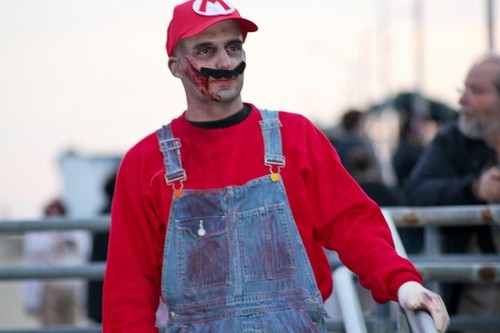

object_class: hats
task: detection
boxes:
[166,0,258,58]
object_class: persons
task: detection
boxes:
[401,57,500,316]
[389,114,427,251]
[331,110,399,206]
[85,170,118,322]
[21,200,92,329]
[100,0,451,333]
